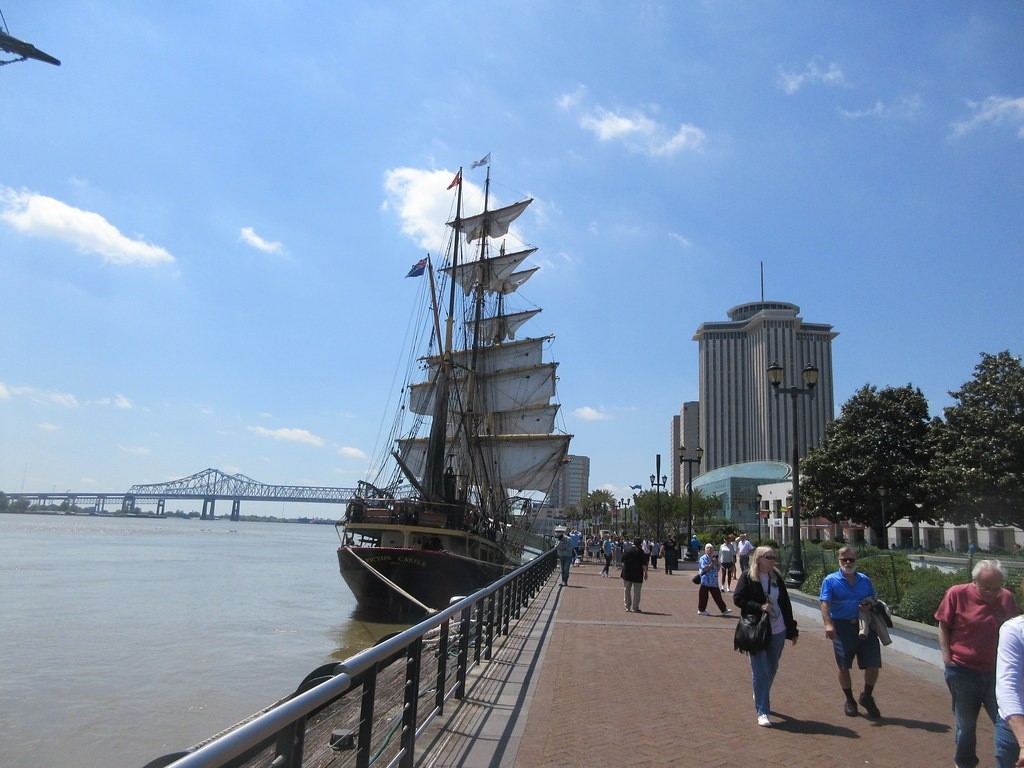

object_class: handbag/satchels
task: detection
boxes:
[734,614,772,652]
[692,574,701,584]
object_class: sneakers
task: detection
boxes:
[758,714,771,725]
[859,693,880,717]
[752,695,755,700]
[845,701,858,715]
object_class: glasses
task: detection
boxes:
[839,559,854,562]
[761,555,776,560]
[977,579,1000,593]
[712,555,719,558]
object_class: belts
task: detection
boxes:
[832,618,859,624]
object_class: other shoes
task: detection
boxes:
[605,576,610,578]
[728,588,732,592]
[697,610,709,615]
[625,609,630,612]
[559,583,568,586]
[721,609,732,615]
[633,610,641,612]
[599,572,604,577]
[721,588,724,592]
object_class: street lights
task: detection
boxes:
[766,359,819,590]
[756,491,762,541]
[612,501,621,535]
[621,497,630,538]
[633,493,643,537]
[677,444,704,562]
[650,474,668,546]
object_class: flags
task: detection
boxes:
[447,173,459,190]
[471,154,488,170]
[405,258,427,278]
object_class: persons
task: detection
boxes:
[598,534,612,578]
[969,539,976,553]
[818,548,882,719]
[718,534,754,593]
[691,535,700,560]
[554,534,573,586]
[569,530,660,569]
[733,546,799,727]
[934,559,1021,768]
[663,533,678,575]
[698,544,732,615]
[620,537,648,613]
[995,613,1024,768]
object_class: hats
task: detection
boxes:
[693,535,696,538]
[558,534,563,541]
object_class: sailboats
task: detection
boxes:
[333,152,574,622]
